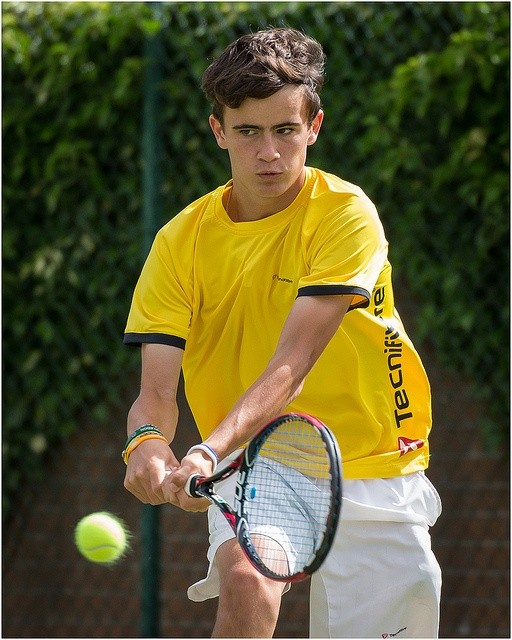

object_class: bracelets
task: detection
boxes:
[185,444,220,474]
[119,424,169,465]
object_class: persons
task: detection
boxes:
[121,28,443,637]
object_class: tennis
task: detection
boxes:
[73,512,128,563]
